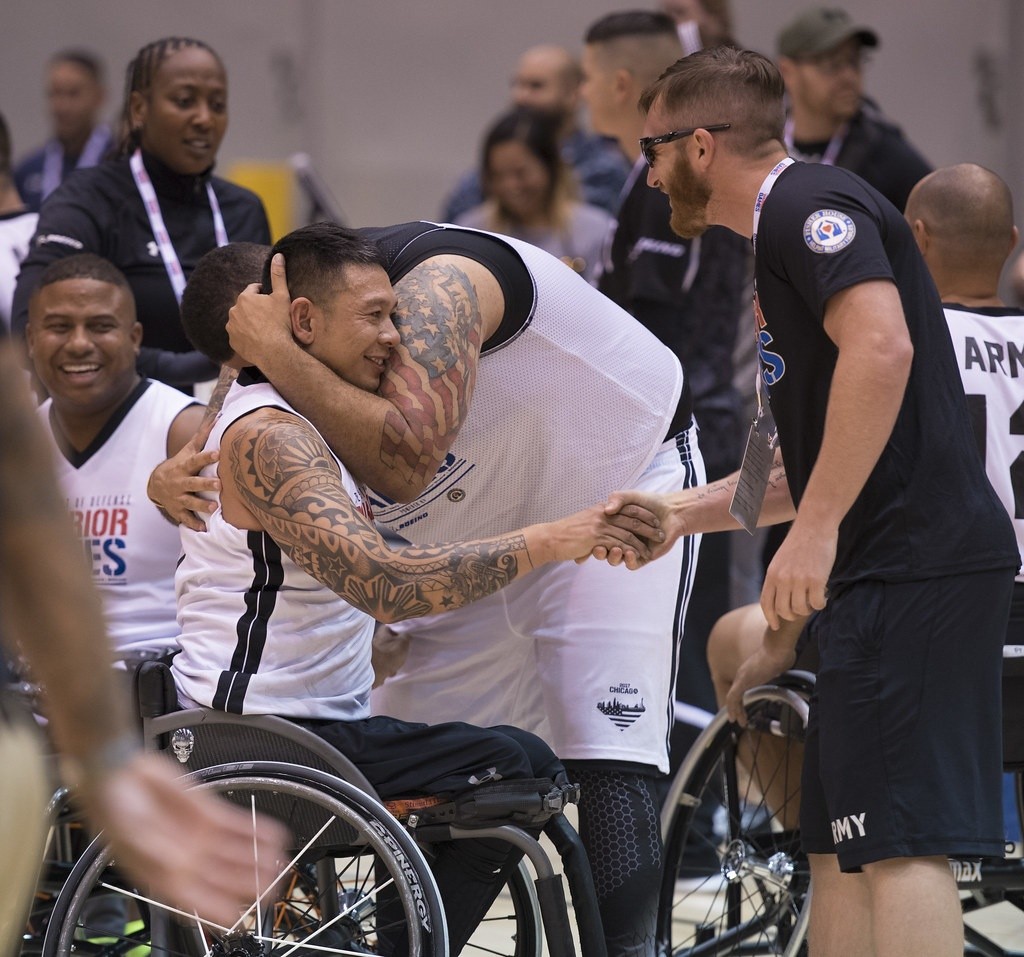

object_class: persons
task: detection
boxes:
[440,0,1024,957]
[0,37,709,957]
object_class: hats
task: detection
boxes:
[781,6,878,58]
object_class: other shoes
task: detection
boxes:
[745,815,808,866]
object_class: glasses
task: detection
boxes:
[640,120,732,167]
[815,52,871,72]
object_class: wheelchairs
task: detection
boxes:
[46,650,606,956]
[653,664,1023,957]
